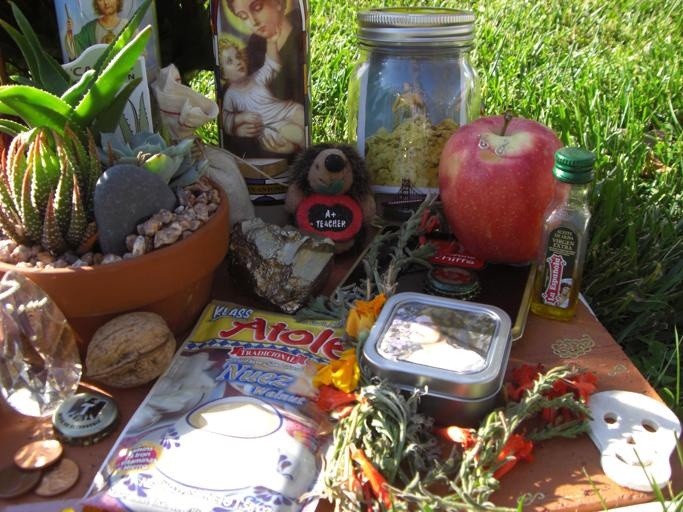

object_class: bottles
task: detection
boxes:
[528,146,597,321]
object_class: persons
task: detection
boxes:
[217,22,306,146]
[63,1,149,63]
[217,0,305,158]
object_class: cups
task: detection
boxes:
[151,396,315,498]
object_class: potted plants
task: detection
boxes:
[0,1,230,344]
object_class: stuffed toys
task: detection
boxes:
[280,140,377,264]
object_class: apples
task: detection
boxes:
[439,115,569,269]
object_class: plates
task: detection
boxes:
[107,423,320,512]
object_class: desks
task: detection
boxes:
[0,155,681,512]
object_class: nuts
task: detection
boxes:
[83,311,177,388]
